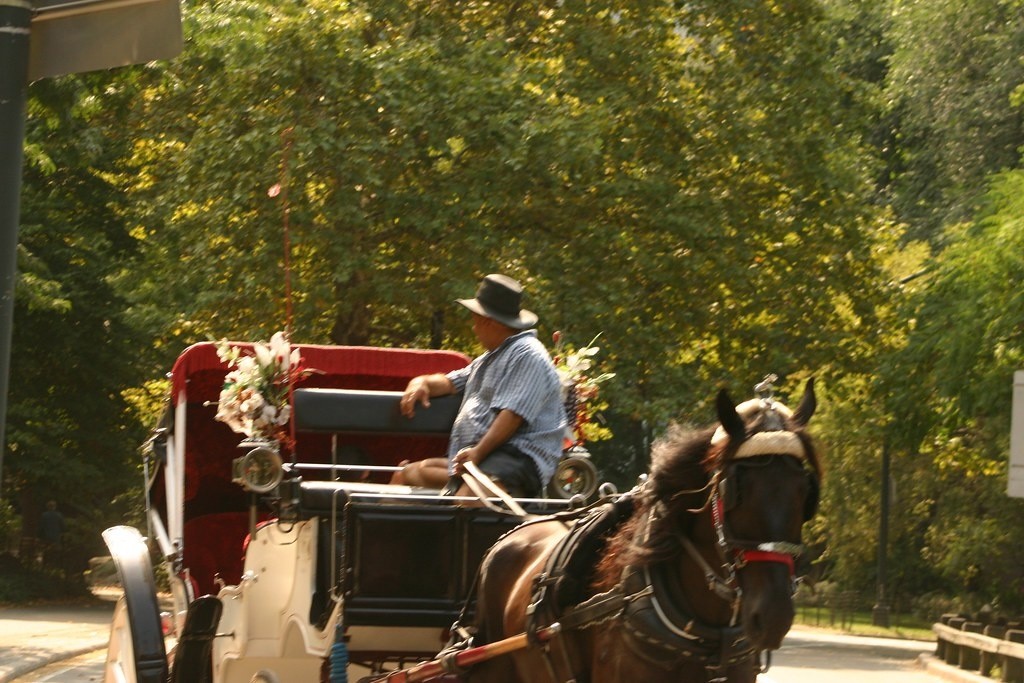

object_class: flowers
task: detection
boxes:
[550,330,620,448]
[206,328,301,454]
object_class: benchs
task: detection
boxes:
[292,387,557,611]
[176,510,276,604]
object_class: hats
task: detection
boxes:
[456,273,539,330]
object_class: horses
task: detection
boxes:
[447,374,828,683]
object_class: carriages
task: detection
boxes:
[101,340,821,683]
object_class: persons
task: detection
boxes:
[333,440,410,503]
[403,275,569,509]
